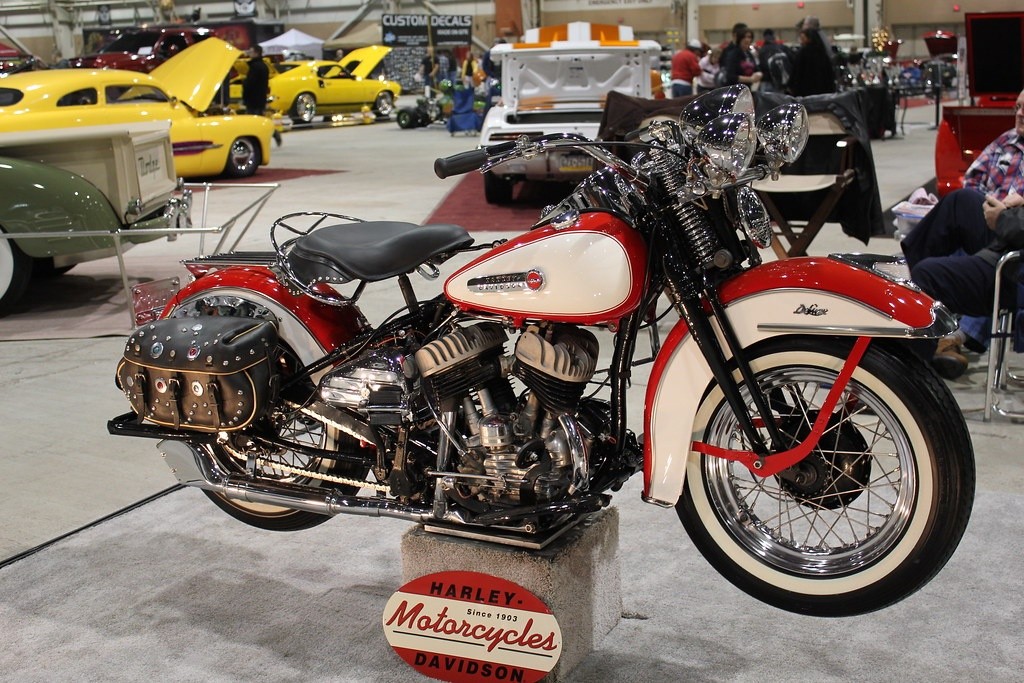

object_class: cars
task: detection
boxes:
[479,20,667,206]
[0,36,282,180]
[0,118,194,317]
[227,45,402,124]
[71,27,216,75]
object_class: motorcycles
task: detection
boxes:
[106,84,978,618]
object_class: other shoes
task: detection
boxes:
[273,130,281,146]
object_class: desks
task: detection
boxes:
[891,83,941,135]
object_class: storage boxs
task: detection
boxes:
[115,317,280,433]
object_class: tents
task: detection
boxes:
[258,23,382,62]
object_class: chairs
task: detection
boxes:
[982,249,1024,422]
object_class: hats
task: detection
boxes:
[688,38,702,48]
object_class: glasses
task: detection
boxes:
[1015,105,1024,112]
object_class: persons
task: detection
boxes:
[336,49,344,62]
[205,44,269,116]
[413,38,508,106]
[670,15,847,98]
[900,89,1024,380]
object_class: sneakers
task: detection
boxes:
[934,335,968,381]
